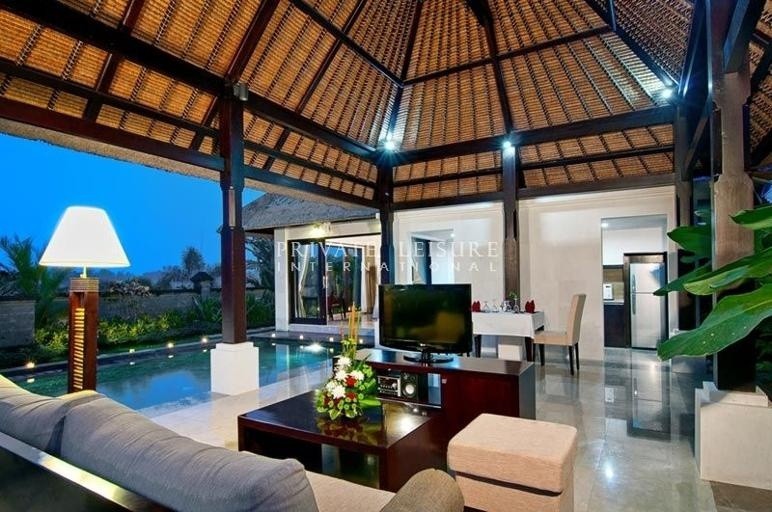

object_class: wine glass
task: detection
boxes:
[482,298,520,313]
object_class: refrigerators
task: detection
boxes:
[629,262,667,350]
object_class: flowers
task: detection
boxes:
[311,301,380,420]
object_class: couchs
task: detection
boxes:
[1,375,465,512]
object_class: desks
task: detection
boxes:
[332,347,536,447]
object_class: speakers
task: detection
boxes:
[238,83,248,101]
[400,371,429,401]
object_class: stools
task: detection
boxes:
[447,414,578,511]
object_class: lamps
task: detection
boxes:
[39,207,131,292]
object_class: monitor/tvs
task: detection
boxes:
[378,283,473,364]
